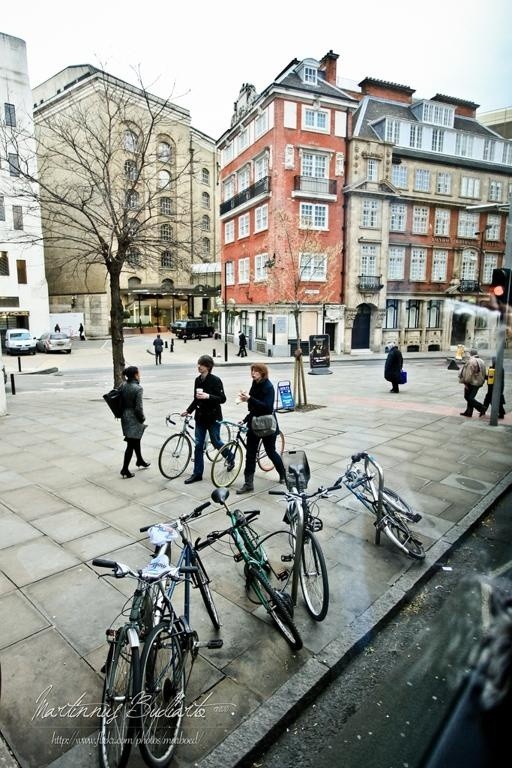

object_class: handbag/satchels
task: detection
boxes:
[103,389,126,418]
[401,371,406,384]
[251,415,277,438]
[156,345,163,352]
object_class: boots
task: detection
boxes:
[236,469,255,494]
[275,462,286,484]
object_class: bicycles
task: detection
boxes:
[158,411,231,478]
[212,417,285,486]
[89,450,426,768]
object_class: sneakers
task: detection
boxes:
[460,405,504,418]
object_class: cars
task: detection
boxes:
[36,331,71,353]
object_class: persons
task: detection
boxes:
[118,366,151,479]
[181,355,235,483]
[238,331,247,356]
[480,357,505,419]
[79,323,85,340]
[384,343,403,393]
[235,363,286,494]
[153,335,163,364]
[55,324,60,332]
[459,348,487,417]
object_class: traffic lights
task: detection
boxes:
[491,268,512,302]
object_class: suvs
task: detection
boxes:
[173,318,214,339]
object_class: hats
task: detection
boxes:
[469,349,477,355]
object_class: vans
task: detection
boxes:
[4,327,36,355]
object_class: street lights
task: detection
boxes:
[474,224,492,304]
[223,259,232,362]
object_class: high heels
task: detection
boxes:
[120,470,135,478]
[136,462,150,467]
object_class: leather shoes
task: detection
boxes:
[185,475,202,484]
[227,455,234,472]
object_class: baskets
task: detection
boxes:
[281,450,311,489]
